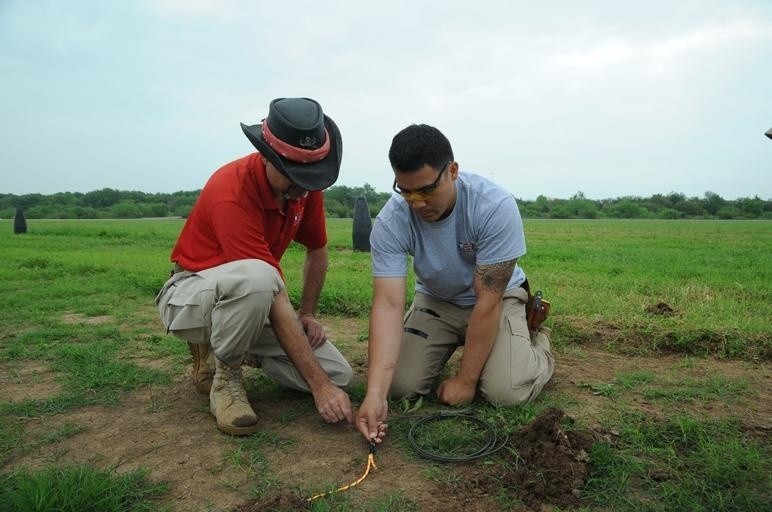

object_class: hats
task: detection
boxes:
[240,96,343,192]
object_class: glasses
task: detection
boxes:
[393,162,450,201]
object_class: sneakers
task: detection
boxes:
[536,326,554,344]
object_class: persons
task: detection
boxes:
[157,96,354,437]
[354,122,558,445]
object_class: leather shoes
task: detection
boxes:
[209,361,259,435]
[189,335,215,394]
